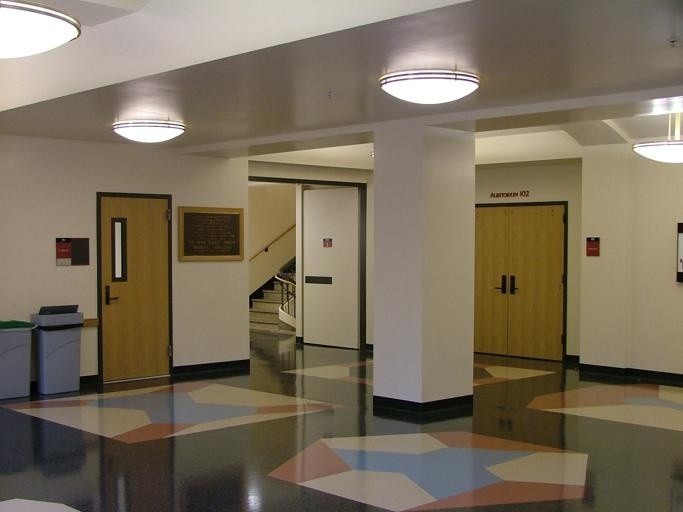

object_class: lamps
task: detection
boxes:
[0,0,81,60]
[112,119,186,144]
[377,68,480,105]
[631,111,683,164]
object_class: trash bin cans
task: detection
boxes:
[30,304,84,394]
[0,320,38,399]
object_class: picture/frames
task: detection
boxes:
[178,206,244,263]
